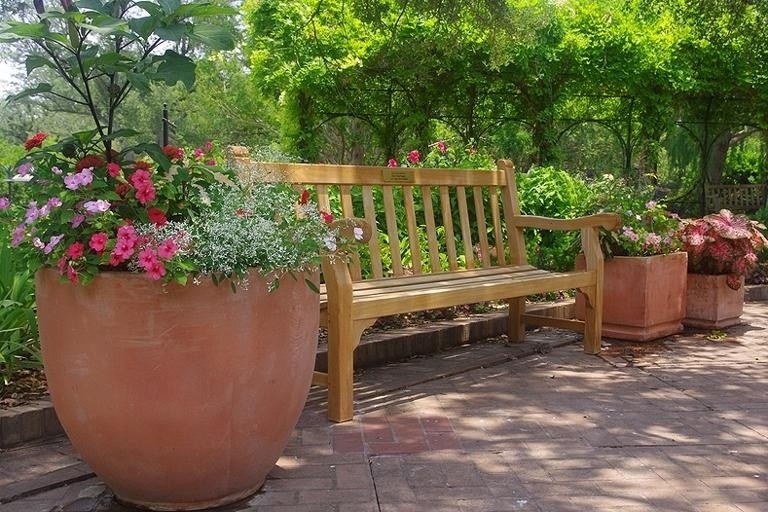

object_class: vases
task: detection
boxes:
[32,260,319,511]
[575,251,746,342]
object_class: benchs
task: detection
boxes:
[223,143,622,424]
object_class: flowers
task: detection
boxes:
[0,128,364,297]
[581,173,768,291]
